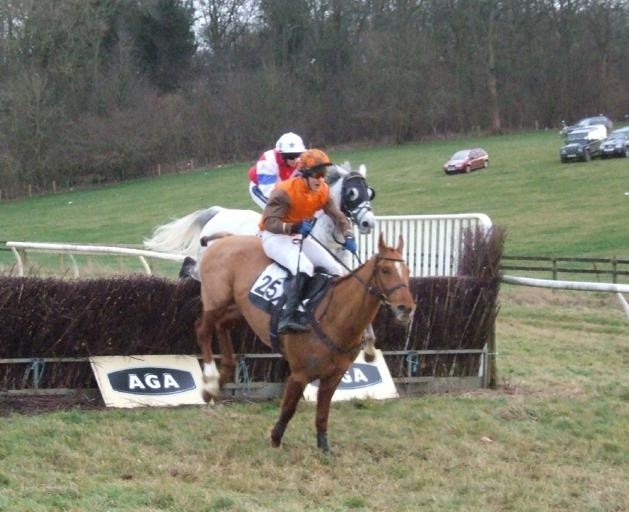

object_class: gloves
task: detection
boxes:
[291,219,311,236]
[343,236,356,253]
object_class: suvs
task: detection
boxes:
[559,116,629,163]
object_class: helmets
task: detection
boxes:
[297,149,332,172]
[276,132,305,153]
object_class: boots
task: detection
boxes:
[278,273,310,333]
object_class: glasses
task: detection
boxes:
[310,168,327,178]
[283,153,299,160]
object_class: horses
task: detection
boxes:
[142,160,377,364]
[160,232,417,455]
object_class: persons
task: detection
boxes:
[247,131,307,215]
[258,146,359,337]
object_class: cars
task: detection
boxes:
[442,148,489,175]
